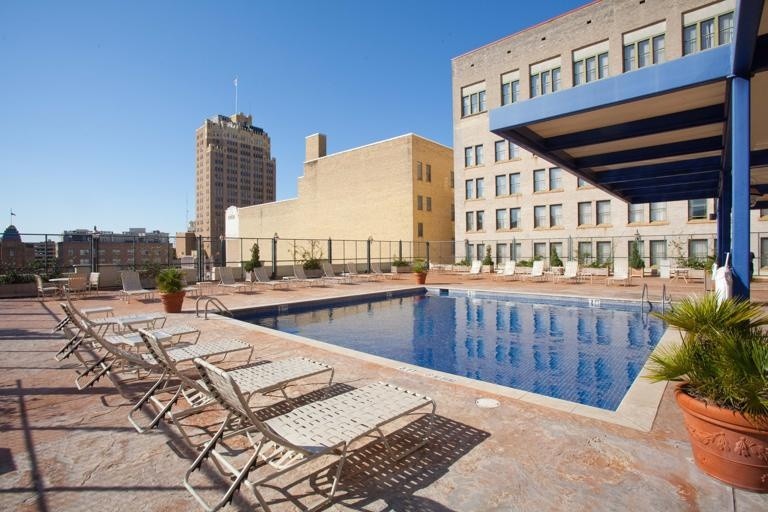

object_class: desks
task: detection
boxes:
[669,268,690,284]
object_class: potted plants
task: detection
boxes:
[703,261,715,291]
[244,243,260,283]
[639,289,768,494]
[481,255,494,273]
[551,248,564,275]
[630,238,645,278]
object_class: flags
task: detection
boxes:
[11,213,16,216]
[232,79,238,86]
